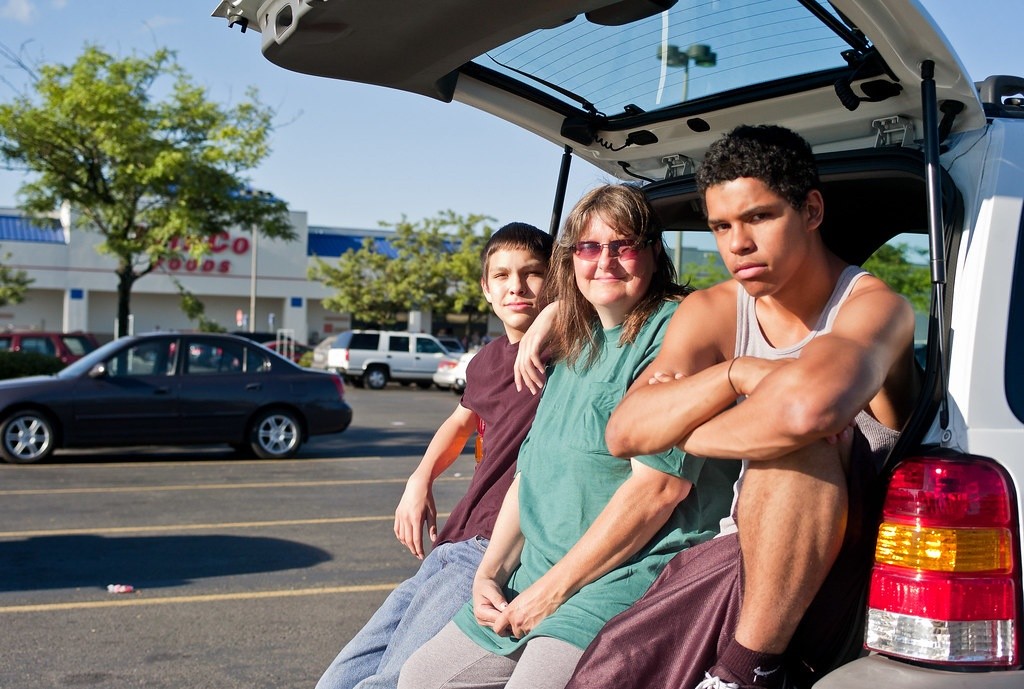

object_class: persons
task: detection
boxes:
[564,124,931,689]
[397,185,741,689]
[313,224,567,689]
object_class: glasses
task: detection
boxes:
[569,239,656,261]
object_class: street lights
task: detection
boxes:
[655,41,718,291]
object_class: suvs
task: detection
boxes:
[202,0,1021,689]
[327,329,458,391]
[1,331,105,376]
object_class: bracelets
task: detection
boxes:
[727,356,743,397]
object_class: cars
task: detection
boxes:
[432,351,482,395]
[230,339,316,370]
[1,329,354,463]
